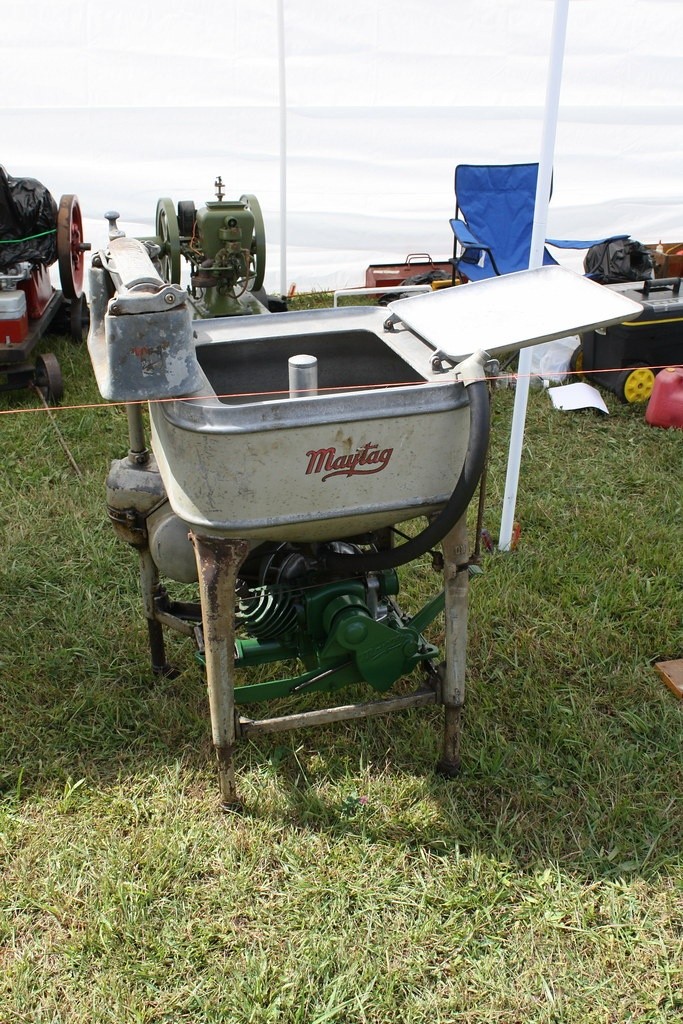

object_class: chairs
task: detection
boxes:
[448,163,631,287]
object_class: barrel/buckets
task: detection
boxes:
[645,367,683,428]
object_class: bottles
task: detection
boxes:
[656,240,665,254]
